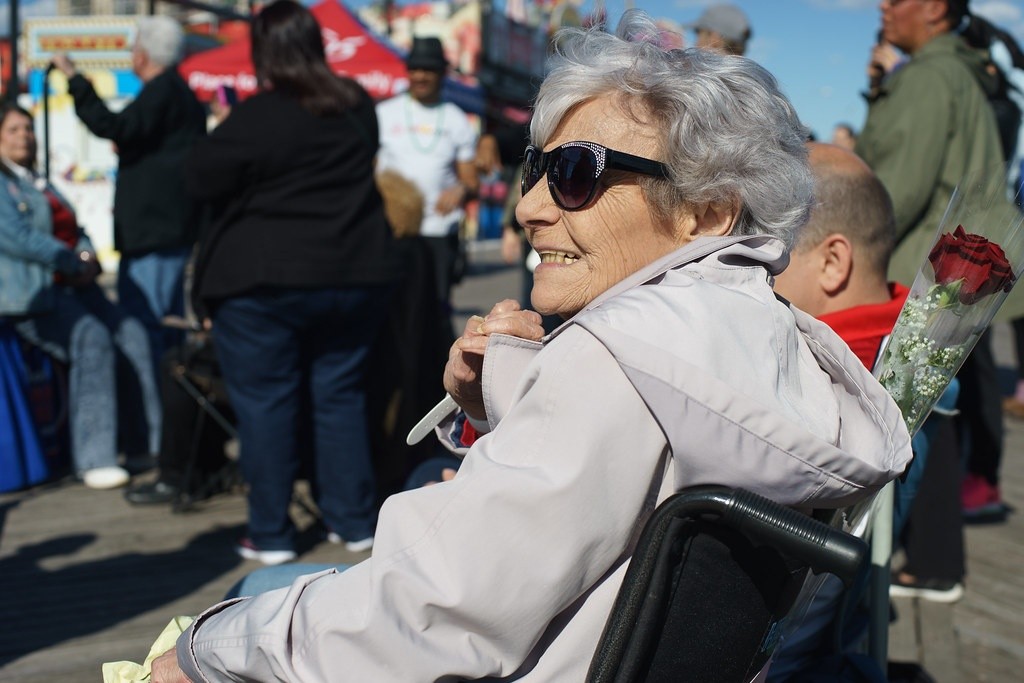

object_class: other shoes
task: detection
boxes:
[963,474,1001,514]
[327,532,374,552]
[83,465,130,490]
[888,567,962,603]
[123,458,236,511]
[233,528,296,564]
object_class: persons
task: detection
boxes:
[158,12,907,683]
[2,1,1024,601]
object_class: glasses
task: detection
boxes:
[520,140,669,211]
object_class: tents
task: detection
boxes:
[170,0,487,114]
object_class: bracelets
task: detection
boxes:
[459,182,473,201]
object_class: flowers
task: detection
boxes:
[880,228,1018,434]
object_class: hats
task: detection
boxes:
[684,3,751,44]
[403,37,448,72]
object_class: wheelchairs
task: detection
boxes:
[580,481,872,683]
